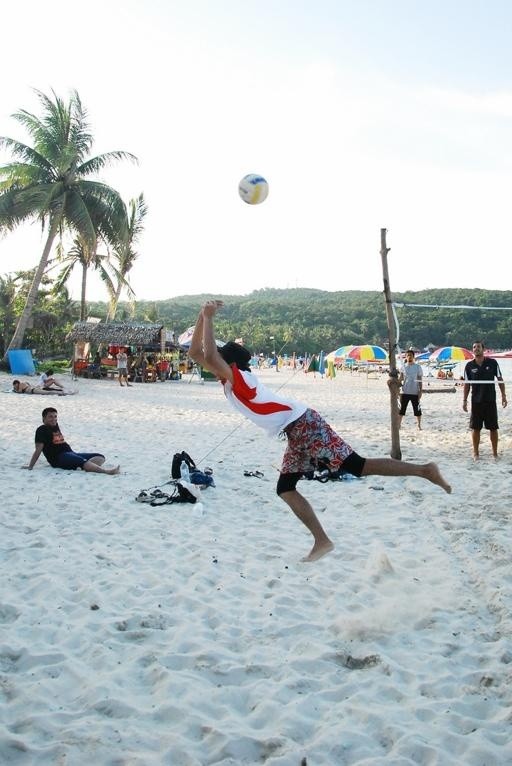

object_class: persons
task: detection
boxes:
[36,370,66,389]
[19,406,121,475]
[396,350,423,430]
[187,299,454,564]
[93,352,104,379]
[116,346,133,387]
[461,341,508,463]
[11,380,64,396]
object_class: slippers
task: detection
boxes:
[136,489,170,506]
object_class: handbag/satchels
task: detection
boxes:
[171,451,196,479]
[190,472,215,489]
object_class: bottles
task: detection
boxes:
[180,460,190,482]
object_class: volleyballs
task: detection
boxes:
[238,173,268,203]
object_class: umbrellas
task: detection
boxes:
[269,343,474,379]
